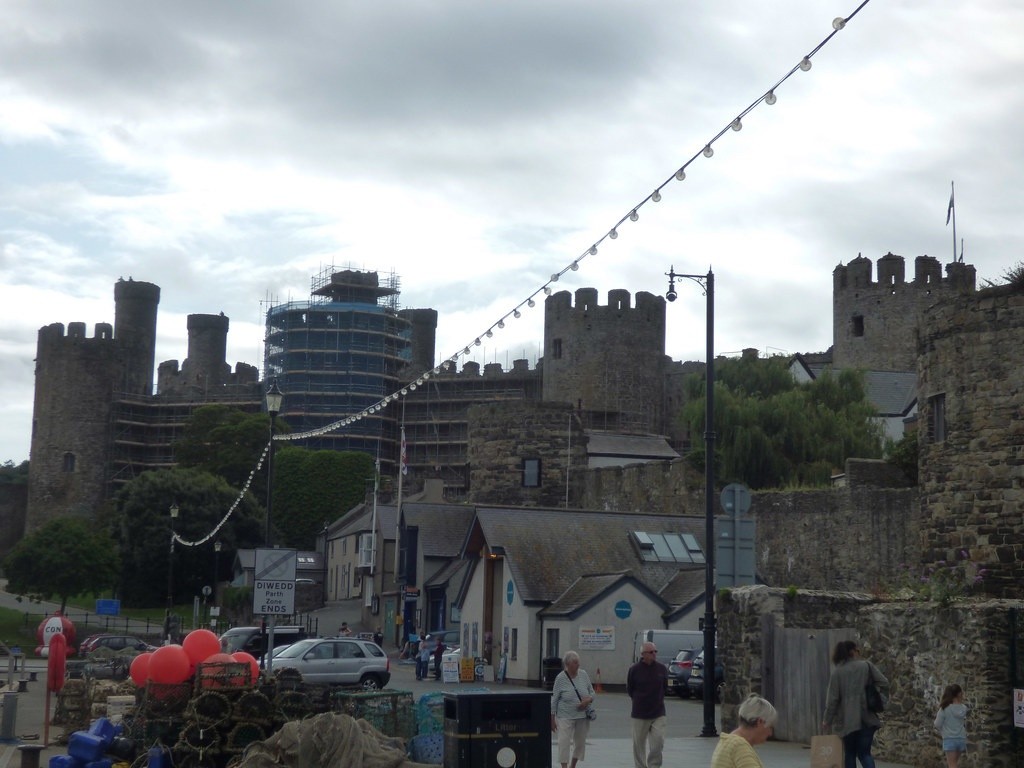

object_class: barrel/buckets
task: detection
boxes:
[50,718,171,768]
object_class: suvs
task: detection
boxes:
[218,625,311,662]
[686,644,723,703]
[667,648,702,699]
[79,633,160,657]
[256,638,391,691]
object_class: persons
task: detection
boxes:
[431,637,444,680]
[415,632,431,680]
[934,684,968,768]
[822,641,890,768]
[710,697,778,768]
[627,642,668,768]
[374,625,383,648]
[551,652,595,768]
[339,622,352,637]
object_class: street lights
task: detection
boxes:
[261,377,285,636]
[164,499,181,640]
[662,262,723,739]
[212,539,225,634]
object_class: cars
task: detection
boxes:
[414,630,461,677]
[254,643,291,667]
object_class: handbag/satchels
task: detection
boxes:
[423,641,429,648]
[586,708,596,720]
[811,733,845,768]
[866,662,884,713]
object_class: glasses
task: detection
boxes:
[645,650,658,654]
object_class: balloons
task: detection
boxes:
[129,630,259,697]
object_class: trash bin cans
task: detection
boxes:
[0,691,16,741]
[542,657,564,691]
[443,692,554,768]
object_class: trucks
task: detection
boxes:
[632,629,705,669]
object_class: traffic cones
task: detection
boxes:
[594,668,603,694]
